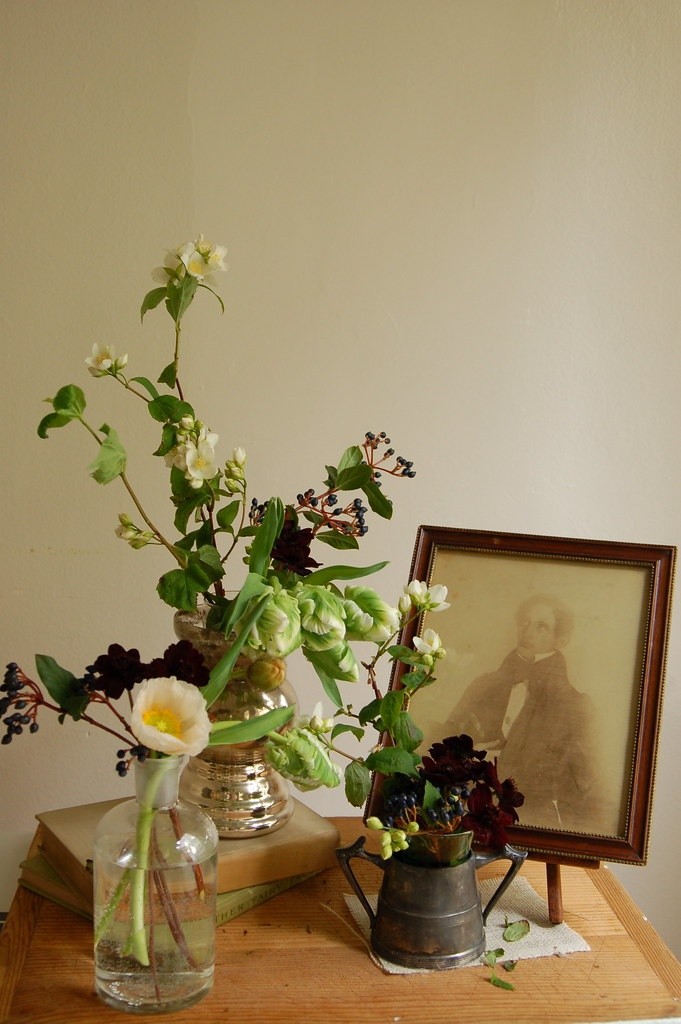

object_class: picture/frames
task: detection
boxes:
[362,523,676,865]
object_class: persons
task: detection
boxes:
[441,591,603,833]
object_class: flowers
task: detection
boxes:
[0,637,209,970]
[367,731,524,844]
[4,212,449,804]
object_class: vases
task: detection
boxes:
[173,589,293,838]
[334,828,529,969]
[89,756,218,1014]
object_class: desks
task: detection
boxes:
[4,812,679,1019]
